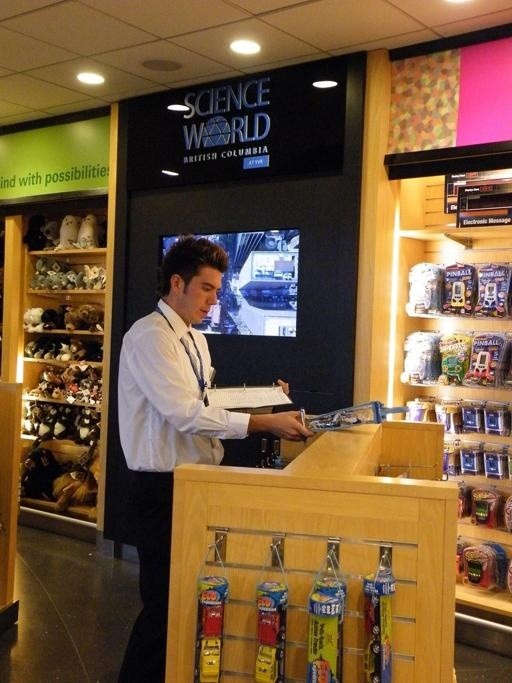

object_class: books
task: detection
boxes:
[202,381,296,415]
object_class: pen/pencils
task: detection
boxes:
[300,408,306,445]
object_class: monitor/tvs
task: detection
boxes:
[157,226,306,345]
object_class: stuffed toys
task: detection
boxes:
[19,211,105,515]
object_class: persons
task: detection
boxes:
[115,238,316,683]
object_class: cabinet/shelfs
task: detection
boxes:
[0,196,127,545]
